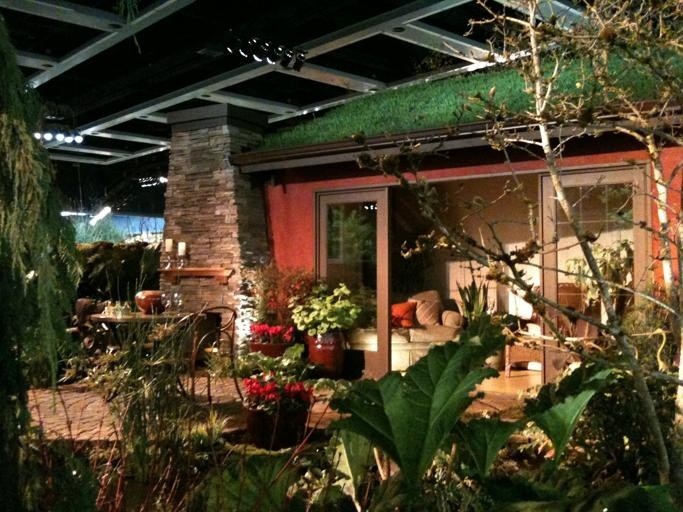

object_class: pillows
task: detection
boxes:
[387,291,445,328]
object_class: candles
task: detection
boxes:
[163,238,186,268]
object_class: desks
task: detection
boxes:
[90,310,194,407]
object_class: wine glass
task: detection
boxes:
[160,291,186,316]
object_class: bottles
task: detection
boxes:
[105,299,131,318]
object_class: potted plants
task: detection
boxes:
[287,282,360,376]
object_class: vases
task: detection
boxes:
[134,289,169,315]
[247,407,307,450]
[248,341,289,358]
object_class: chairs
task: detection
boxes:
[169,303,243,403]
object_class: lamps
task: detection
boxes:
[41,127,83,145]
[225,36,305,74]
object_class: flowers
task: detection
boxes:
[248,322,293,342]
[243,371,313,408]
